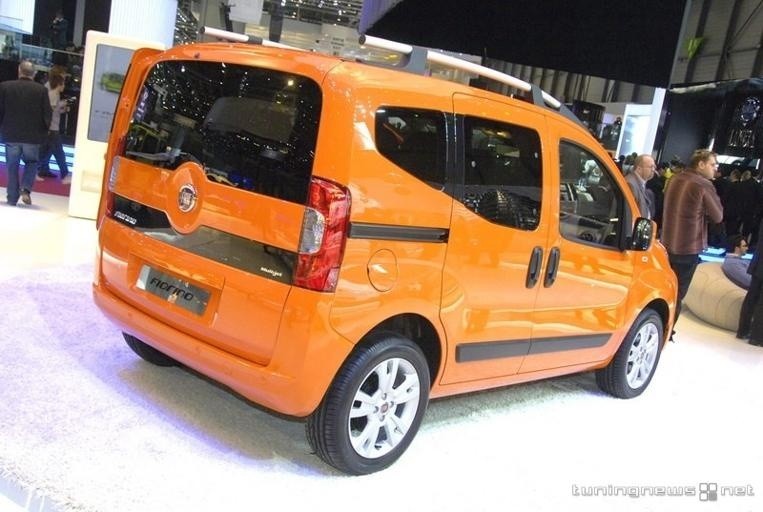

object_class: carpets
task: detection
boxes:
[0,160,72,199]
[1,278,611,512]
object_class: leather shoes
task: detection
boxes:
[735,331,763,347]
[19,188,32,205]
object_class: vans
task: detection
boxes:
[91,25,680,477]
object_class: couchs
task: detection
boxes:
[680,259,757,339]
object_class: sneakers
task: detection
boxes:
[29,170,71,185]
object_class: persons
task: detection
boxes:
[723,233,753,288]
[733,208,762,348]
[659,146,724,345]
[613,149,761,252]
[0,10,93,205]
[581,159,603,185]
